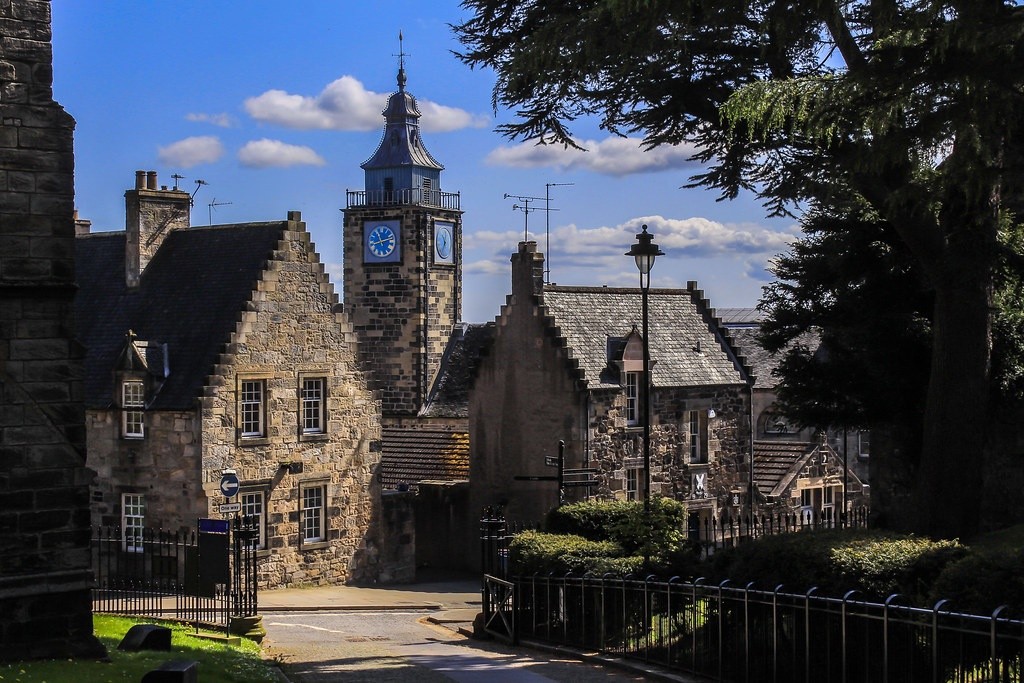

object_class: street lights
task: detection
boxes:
[624,223,666,631]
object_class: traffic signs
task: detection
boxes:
[563,480,601,487]
[563,468,598,475]
[545,456,559,467]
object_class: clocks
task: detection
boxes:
[436,227,453,259]
[367,226,397,259]
